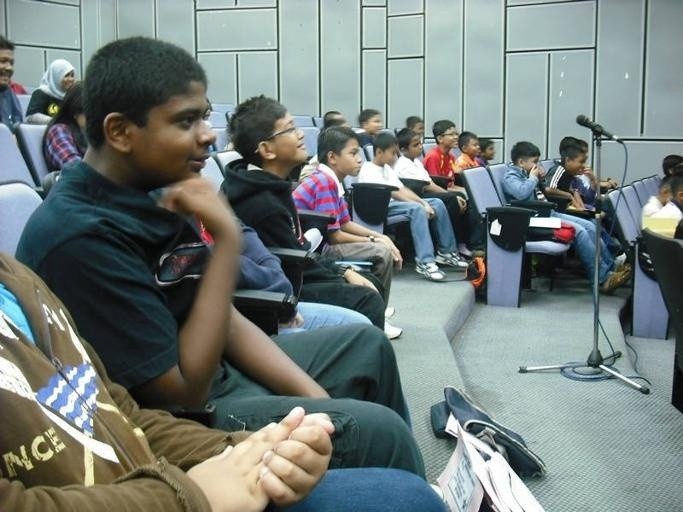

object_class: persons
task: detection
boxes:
[0,248,450,511]
[202,95,682,339]
[1,36,91,173]
[14,38,427,479]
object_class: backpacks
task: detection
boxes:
[528,218,576,243]
[427,384,552,476]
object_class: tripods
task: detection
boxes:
[516,144,651,396]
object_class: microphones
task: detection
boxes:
[576,113,624,145]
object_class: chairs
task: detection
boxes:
[619,184,643,234]
[216,149,335,254]
[209,112,228,127]
[540,159,555,173]
[210,104,234,113]
[642,226,682,415]
[460,165,571,307]
[46,172,317,335]
[351,127,395,136]
[314,116,324,128]
[363,143,454,198]
[300,126,321,155]
[17,95,31,122]
[0,123,37,189]
[199,154,373,267]
[293,115,313,129]
[226,111,235,124]
[13,124,51,186]
[342,146,430,235]
[632,180,648,207]
[487,163,574,244]
[210,127,228,152]
[423,143,436,157]
[452,149,461,157]
[605,188,669,340]
[0,181,298,428]
[642,174,662,196]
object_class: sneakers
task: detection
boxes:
[384,321,403,338]
[457,241,474,255]
[434,253,466,268]
[614,250,627,266]
[599,266,635,296]
[384,305,397,319]
[413,261,444,279]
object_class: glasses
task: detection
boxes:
[250,121,301,154]
[438,130,458,137]
